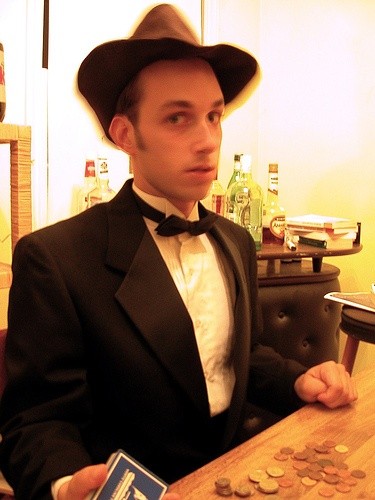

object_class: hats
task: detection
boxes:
[77,4,261,145]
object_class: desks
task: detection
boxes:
[159,371,375,500]
[241,244,363,444]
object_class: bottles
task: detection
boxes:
[205,169,225,218]
[226,154,241,221]
[87,158,116,209]
[264,163,285,246]
[77,160,98,214]
[231,156,262,251]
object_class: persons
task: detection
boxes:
[1,4,359,500]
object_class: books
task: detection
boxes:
[286,214,358,249]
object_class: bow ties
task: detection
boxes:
[153,211,219,237]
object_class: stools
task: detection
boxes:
[340,305,375,378]
